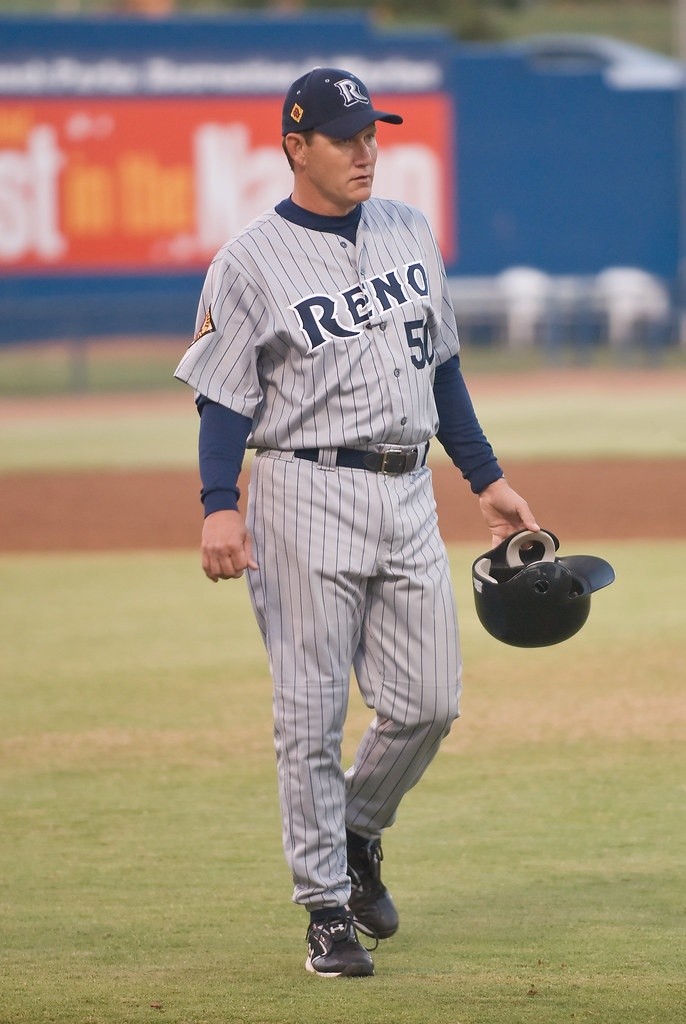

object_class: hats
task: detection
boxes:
[277,68,405,140]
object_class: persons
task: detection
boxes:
[173,68,541,978]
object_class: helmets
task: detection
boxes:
[468,525,620,649]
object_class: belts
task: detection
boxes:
[292,436,431,476]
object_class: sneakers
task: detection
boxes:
[302,903,381,979]
[344,830,402,939]
